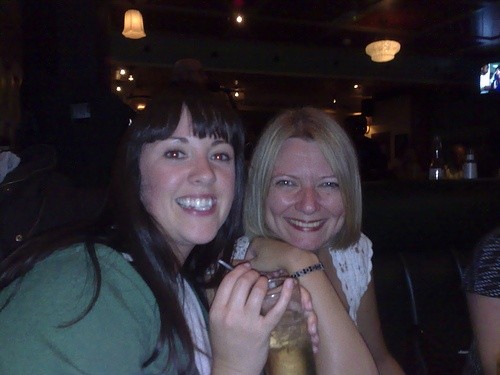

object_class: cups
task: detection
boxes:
[260,277,317,375]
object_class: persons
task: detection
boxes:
[231,107,403,375]
[0,90,319,375]
[462,226,500,375]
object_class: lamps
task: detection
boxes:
[365,17,401,62]
[122,9,146,40]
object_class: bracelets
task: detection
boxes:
[291,263,323,278]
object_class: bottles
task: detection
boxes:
[427,136,445,180]
[464,148,478,180]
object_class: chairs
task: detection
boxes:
[398,248,472,374]
